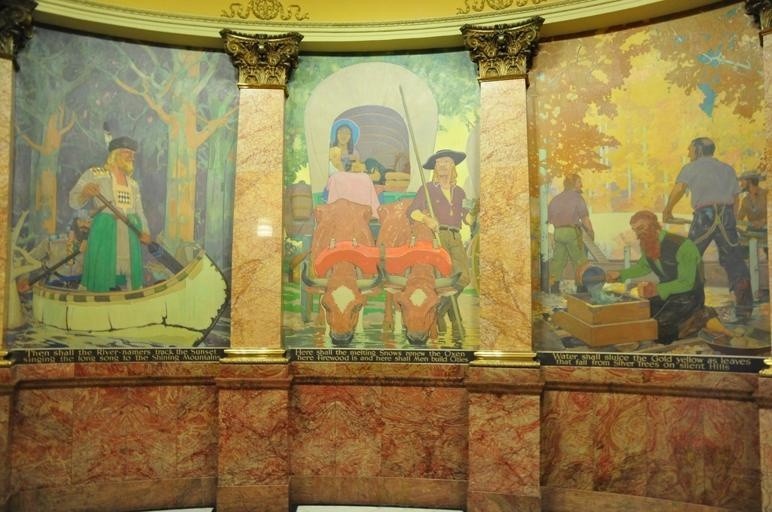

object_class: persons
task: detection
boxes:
[327,119,381,218]
[606,211,719,345]
[547,173,594,289]
[68,136,152,292]
[407,149,481,333]
[663,137,753,321]
[736,171,767,230]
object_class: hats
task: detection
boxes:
[421,147,468,170]
[330,118,361,146]
[735,169,767,181]
[109,135,138,153]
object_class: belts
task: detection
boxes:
[439,224,460,233]
[553,223,576,229]
